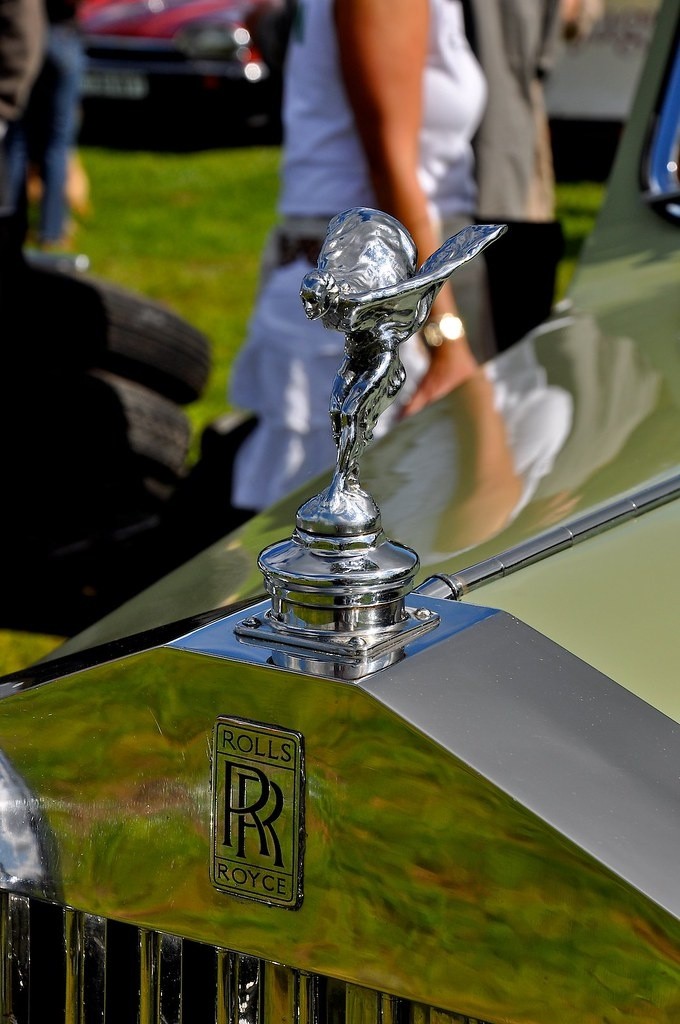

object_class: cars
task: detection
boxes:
[49,1,299,154]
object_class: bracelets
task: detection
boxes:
[420,312,467,351]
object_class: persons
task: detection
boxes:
[0,0,88,258]
[233,0,490,513]
